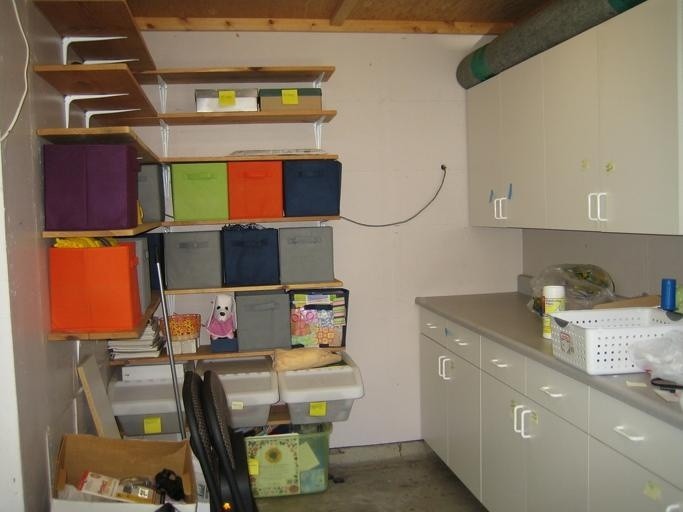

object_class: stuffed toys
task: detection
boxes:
[199,295,237,341]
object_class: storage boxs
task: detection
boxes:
[275,348,363,423]
[236,422,334,498]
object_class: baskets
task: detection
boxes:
[159,314,201,341]
[549,307,683,376]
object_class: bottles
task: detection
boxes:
[539,284,565,342]
[660,278,676,312]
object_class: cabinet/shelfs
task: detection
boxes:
[586,388,681,512]
[541,0,681,236]
[478,334,585,512]
[417,304,480,503]
[465,49,541,228]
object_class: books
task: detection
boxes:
[105,315,165,362]
